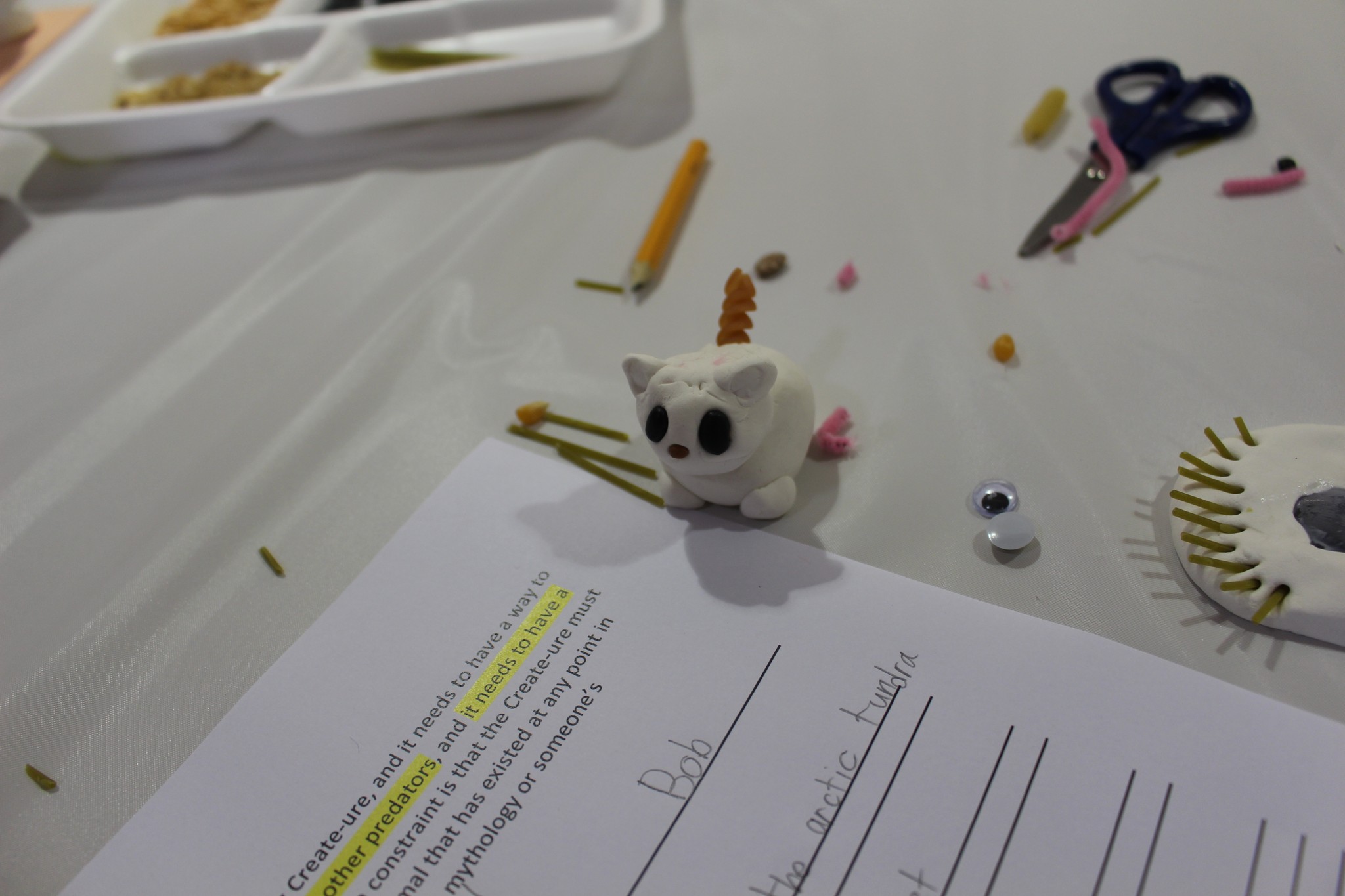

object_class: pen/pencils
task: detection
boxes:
[627,139,709,290]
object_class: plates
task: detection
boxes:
[2,0,673,157]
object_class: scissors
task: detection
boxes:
[1018,56,1255,257]
